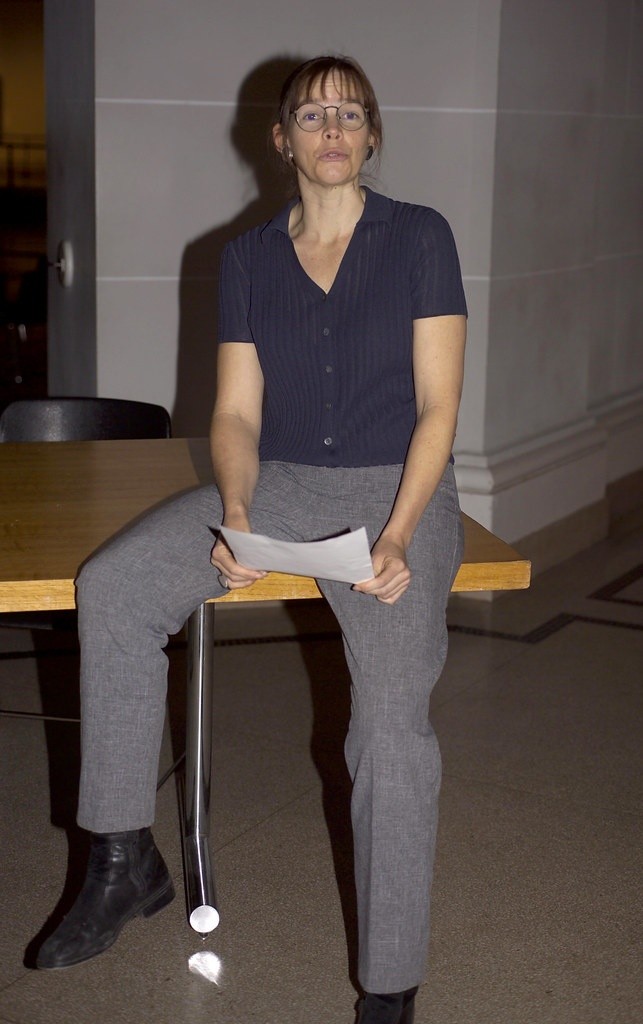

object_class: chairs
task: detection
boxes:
[2,399,186,796]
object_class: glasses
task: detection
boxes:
[286,101,370,132]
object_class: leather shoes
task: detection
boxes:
[35,830,177,971]
[354,985,418,1023]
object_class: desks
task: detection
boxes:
[0,437,533,936]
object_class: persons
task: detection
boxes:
[32,54,473,1024]
